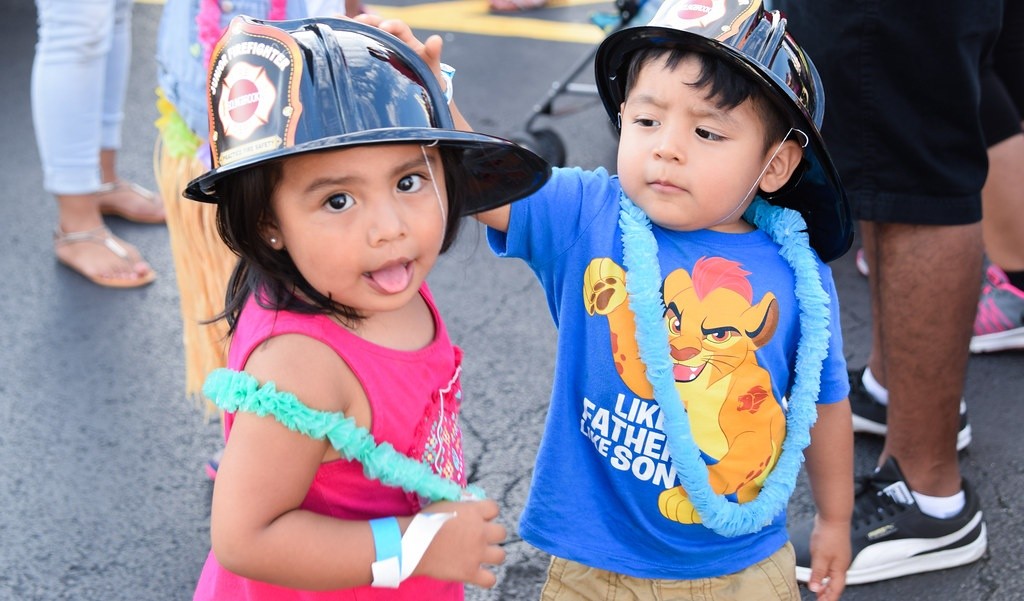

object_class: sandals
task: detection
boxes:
[98,179,166,223]
[52,222,155,286]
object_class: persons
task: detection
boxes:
[350,1,856,600]
[153,0,366,480]
[759,0,1024,586]
[28,0,173,290]
[189,12,554,601]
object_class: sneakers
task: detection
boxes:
[790,454,988,584]
[969,263,1024,353]
[840,367,972,451]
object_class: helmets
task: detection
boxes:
[595,0,825,198]
[183,13,553,217]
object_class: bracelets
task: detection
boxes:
[440,60,456,103]
[367,505,457,589]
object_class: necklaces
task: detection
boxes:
[600,171,831,539]
